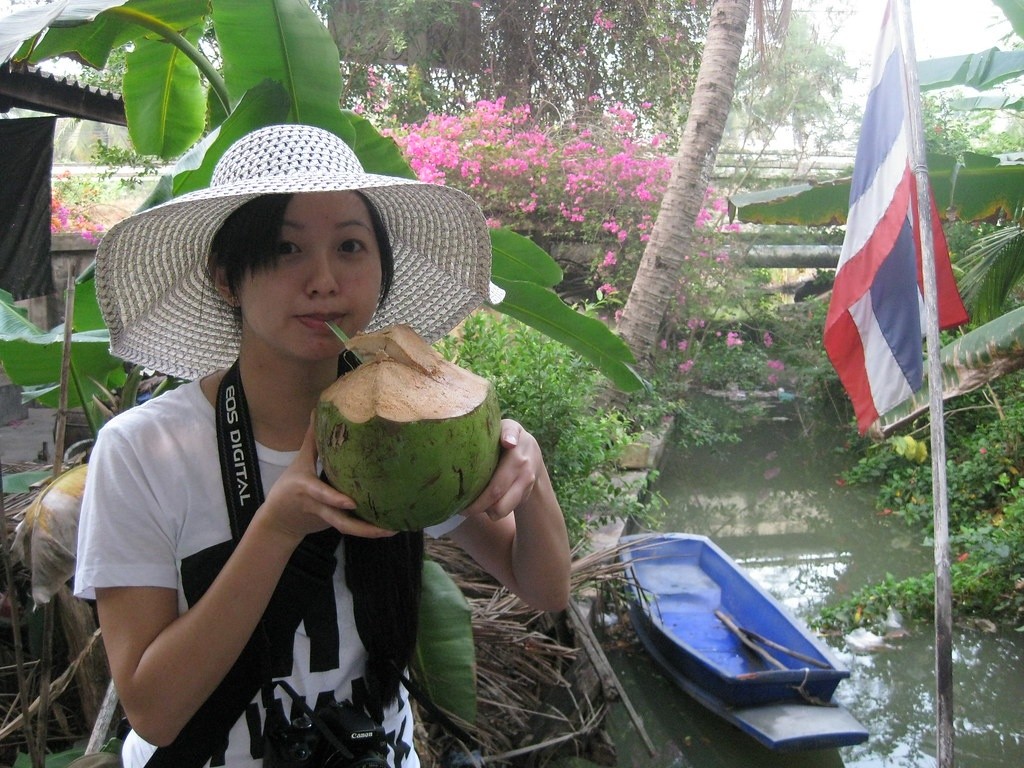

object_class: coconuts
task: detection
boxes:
[314,322,502,528]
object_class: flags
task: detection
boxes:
[823,1,970,436]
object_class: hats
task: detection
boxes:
[95,124,489,381]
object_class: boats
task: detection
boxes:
[618,532,870,753]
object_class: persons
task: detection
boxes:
[71,124,572,768]
